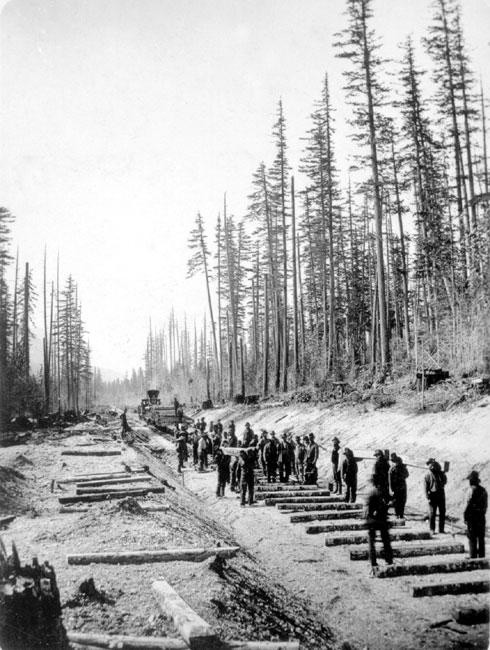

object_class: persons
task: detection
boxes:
[390,456,410,519]
[424,458,448,535]
[329,437,343,495]
[119,408,129,440]
[176,404,184,423]
[340,446,363,502]
[462,471,489,560]
[362,473,396,568]
[174,398,180,414]
[369,449,394,519]
[174,415,319,506]
